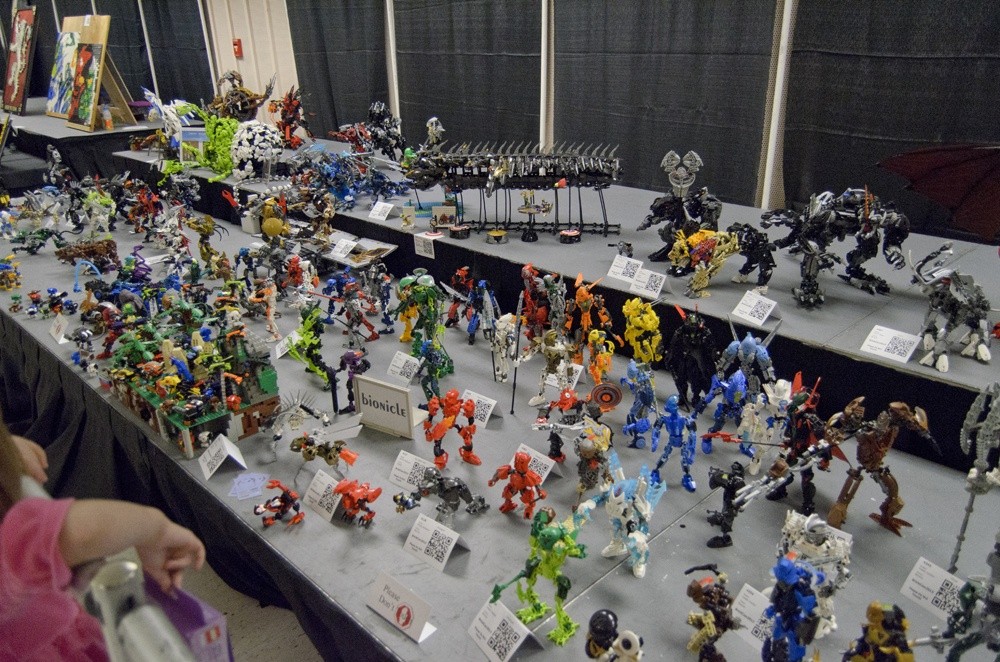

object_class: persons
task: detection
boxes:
[0,415,205,662]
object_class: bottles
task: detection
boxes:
[101,104,114,130]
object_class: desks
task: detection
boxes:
[0,99,999,662]
